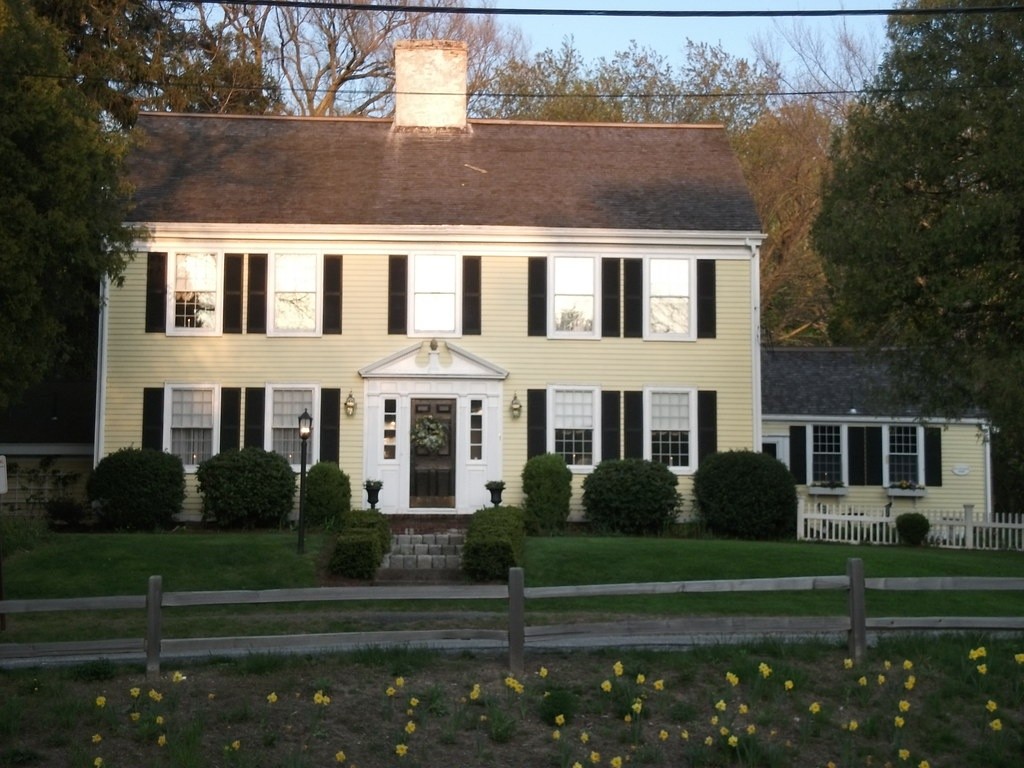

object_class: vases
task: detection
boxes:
[808,487,847,496]
[887,488,926,498]
[425,446,441,458]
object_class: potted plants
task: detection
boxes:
[484,480,506,504]
[362,479,384,505]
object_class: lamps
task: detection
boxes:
[344,391,357,416]
[510,394,523,419]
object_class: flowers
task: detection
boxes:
[410,415,452,449]
[811,480,846,488]
[889,480,925,490]
